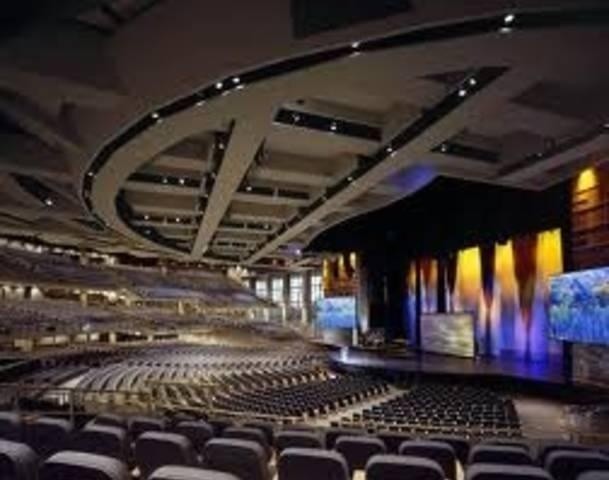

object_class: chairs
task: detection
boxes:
[1,388,608,480]
